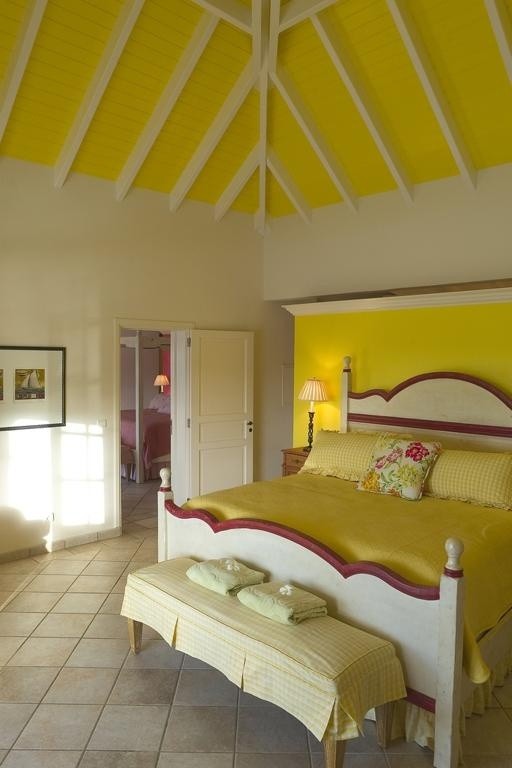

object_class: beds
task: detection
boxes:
[119,409,171,483]
[158,356,512,767]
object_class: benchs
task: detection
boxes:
[123,557,407,768]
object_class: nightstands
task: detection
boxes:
[281,446,309,478]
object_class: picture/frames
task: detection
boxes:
[1,346,66,430]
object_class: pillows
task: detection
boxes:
[297,429,512,512]
[150,394,172,414]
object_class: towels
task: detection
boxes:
[186,557,327,625]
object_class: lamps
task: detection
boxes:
[299,379,329,451]
[154,375,169,394]
